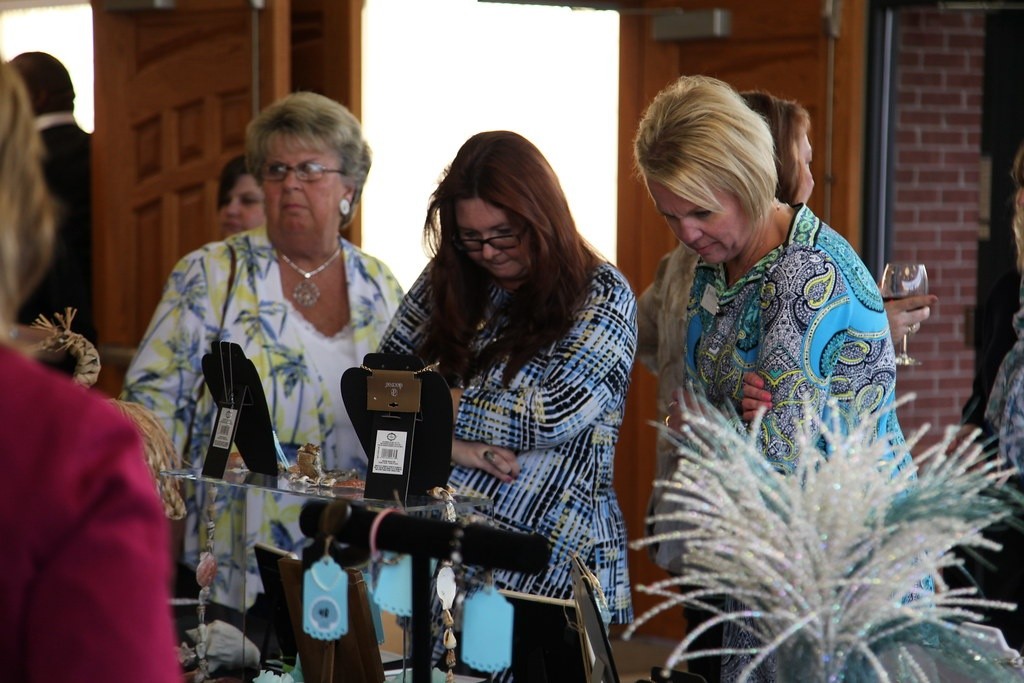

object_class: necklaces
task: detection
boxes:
[278,245,339,304]
[356,359,442,377]
[218,339,235,410]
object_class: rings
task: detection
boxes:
[908,326,912,334]
[483,451,494,460]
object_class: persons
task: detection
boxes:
[121,94,406,622]
[375,131,639,682]
[215,153,267,238]
[946,149,1023,651]
[636,92,938,682]
[633,75,935,683]
[8,51,96,341]
[0,55,180,683]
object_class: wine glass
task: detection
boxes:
[880,262,929,365]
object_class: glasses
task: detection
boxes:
[446,221,531,252]
[260,160,346,182]
